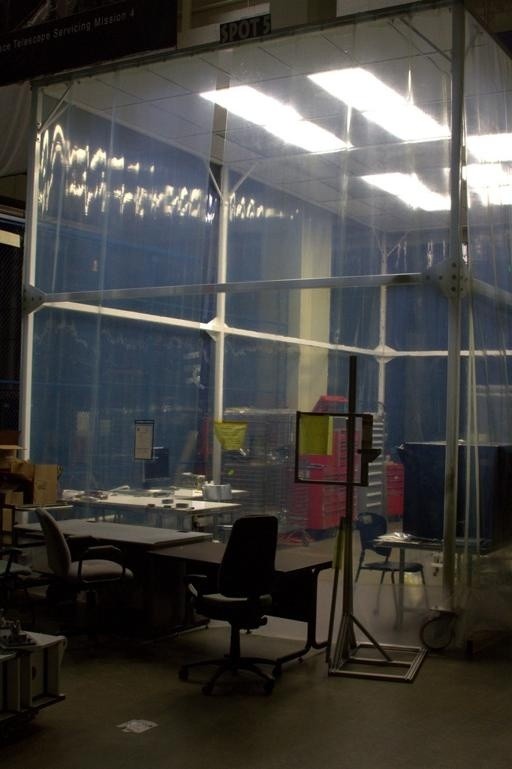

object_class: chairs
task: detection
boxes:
[0,488,286,698]
[354,508,433,633]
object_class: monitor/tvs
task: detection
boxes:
[142,446,170,479]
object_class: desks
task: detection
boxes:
[0,623,67,722]
[143,542,333,676]
[371,535,511,661]
